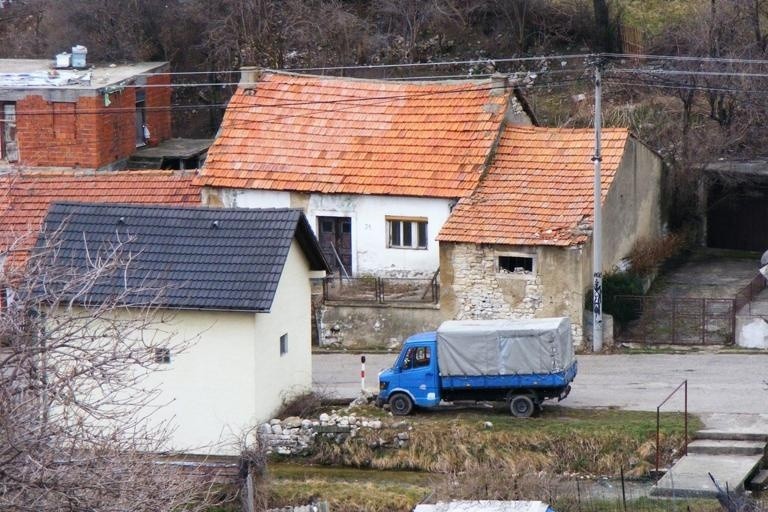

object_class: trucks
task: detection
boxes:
[378,317,578,420]
